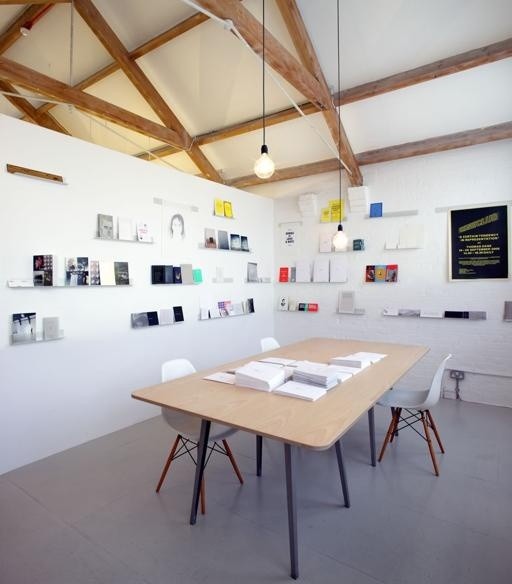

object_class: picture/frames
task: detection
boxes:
[449,204,511,283]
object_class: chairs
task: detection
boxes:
[374,353,452,477]
[260,337,282,353]
[156,357,245,515]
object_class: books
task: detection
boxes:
[165,267,174,282]
[205,352,388,401]
[152,265,164,283]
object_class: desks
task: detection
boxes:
[130,335,431,580]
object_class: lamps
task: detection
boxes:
[253,0,276,179]
[19,20,34,37]
[332,0,349,250]
